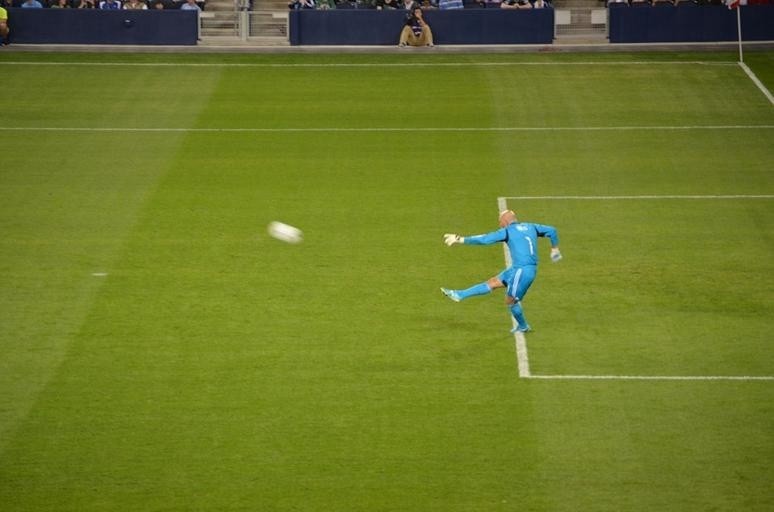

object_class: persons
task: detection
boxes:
[439,209,563,334]
[398,7,433,47]
[0,1,201,48]
[607,1,748,8]
[287,0,550,9]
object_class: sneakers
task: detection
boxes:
[394,42,437,50]
[510,325,533,334]
[439,286,461,303]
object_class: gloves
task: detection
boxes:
[443,233,466,247]
[550,247,563,263]
